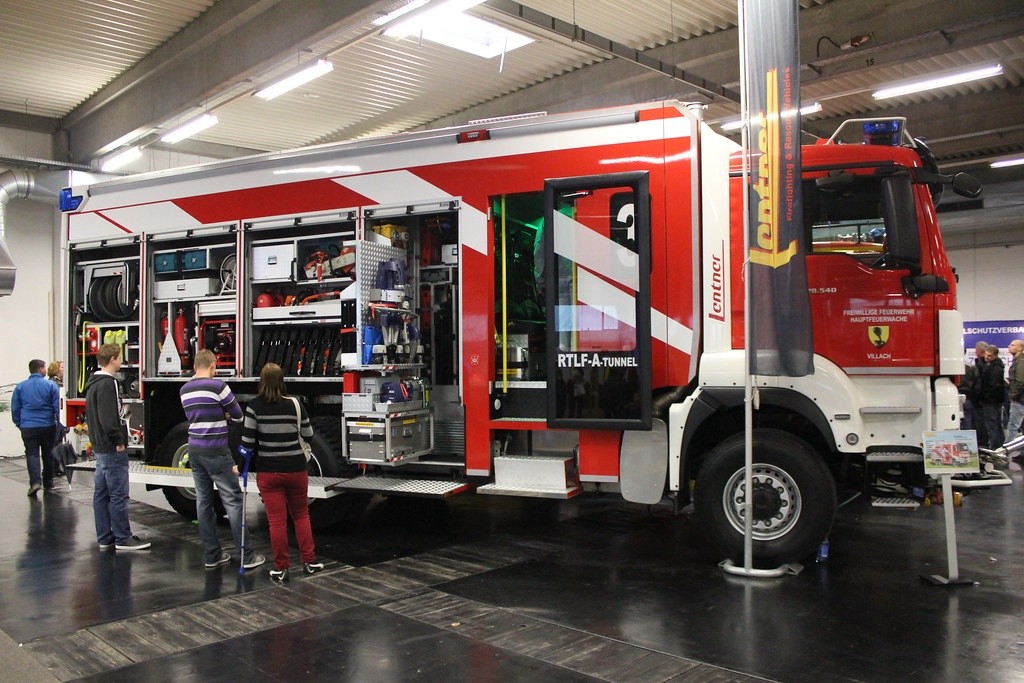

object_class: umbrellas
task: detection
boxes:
[52,435,77,491]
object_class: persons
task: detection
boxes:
[533,192,574,351]
[179,349,265,568]
[233,363,325,579]
[958,340,1024,460]
[84,345,151,549]
[10,359,59,495]
[46,360,64,476]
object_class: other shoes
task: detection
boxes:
[1012,455,1024,459]
[28,483,41,496]
[54,468,64,474]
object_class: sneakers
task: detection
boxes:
[205,553,231,566]
[243,554,265,568]
[269,568,288,580]
[303,562,324,575]
[115,535,151,550]
[99,542,113,548]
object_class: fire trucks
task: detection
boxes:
[60,99,983,565]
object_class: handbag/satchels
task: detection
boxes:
[964,391,977,411]
[297,434,312,463]
